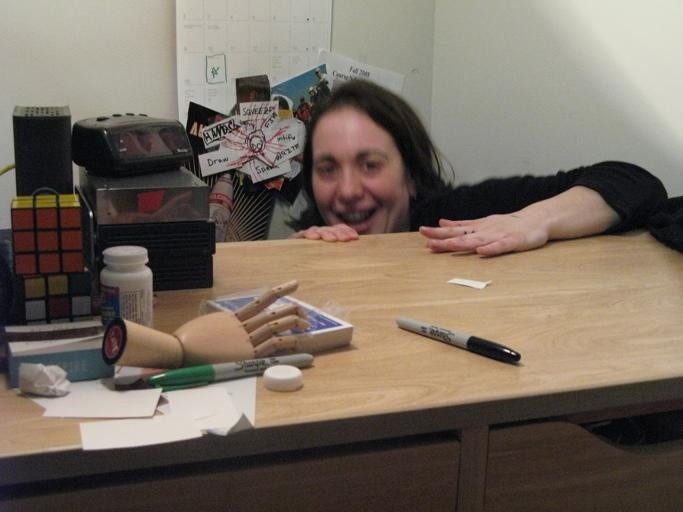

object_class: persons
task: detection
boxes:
[287,79,667,260]
[293,67,338,126]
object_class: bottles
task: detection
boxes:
[99,245,153,331]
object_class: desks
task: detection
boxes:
[0,231,681,511]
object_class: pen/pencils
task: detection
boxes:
[395,314,522,364]
[149,352,316,393]
